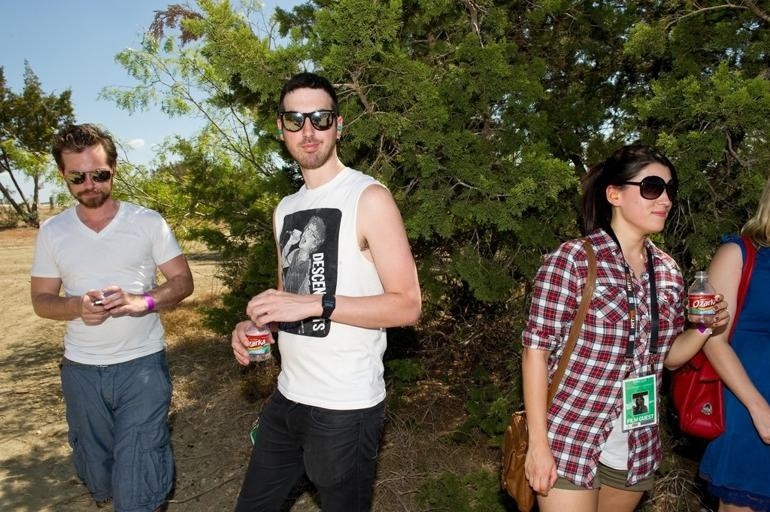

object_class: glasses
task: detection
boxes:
[280,110,335,131]
[62,169,113,183]
[624,175,675,202]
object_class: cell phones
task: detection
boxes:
[94,299,116,310]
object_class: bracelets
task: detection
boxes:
[142,292,155,315]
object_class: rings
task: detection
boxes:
[725,306,730,316]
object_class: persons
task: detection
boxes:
[520,143,732,512]
[701,178,770,511]
[280,215,328,332]
[632,396,647,415]
[31,122,194,511]
[229,72,422,512]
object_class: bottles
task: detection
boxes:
[245,318,271,363]
[686,271,717,325]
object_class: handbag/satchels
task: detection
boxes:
[500,410,536,511]
[671,349,725,440]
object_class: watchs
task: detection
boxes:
[322,292,336,320]
